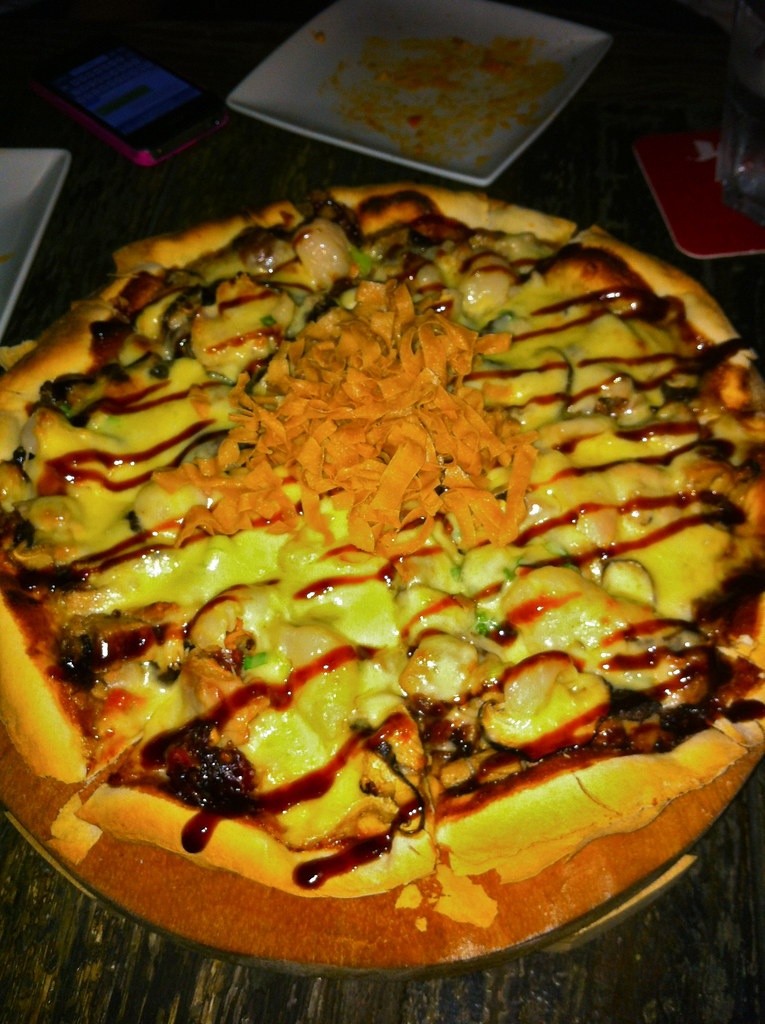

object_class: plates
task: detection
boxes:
[224,0,615,190]
[0,145,72,347]
[1,698,765,977]
[633,129,765,260]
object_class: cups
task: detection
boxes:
[716,85,765,225]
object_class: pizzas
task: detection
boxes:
[0,183,764,975]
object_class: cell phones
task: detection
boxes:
[27,41,230,167]
